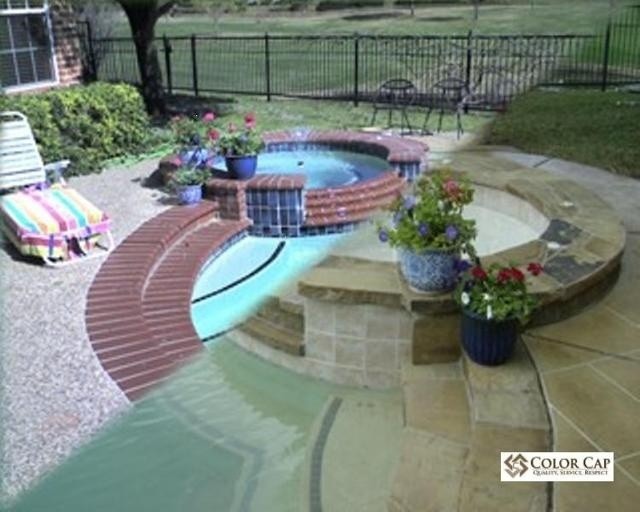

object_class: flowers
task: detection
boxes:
[370,166,544,317]
[203,112,268,158]
[164,155,209,188]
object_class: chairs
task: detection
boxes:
[0,110,115,266]
[369,77,472,142]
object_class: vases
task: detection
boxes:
[174,185,203,205]
[455,307,519,366]
[223,155,257,177]
